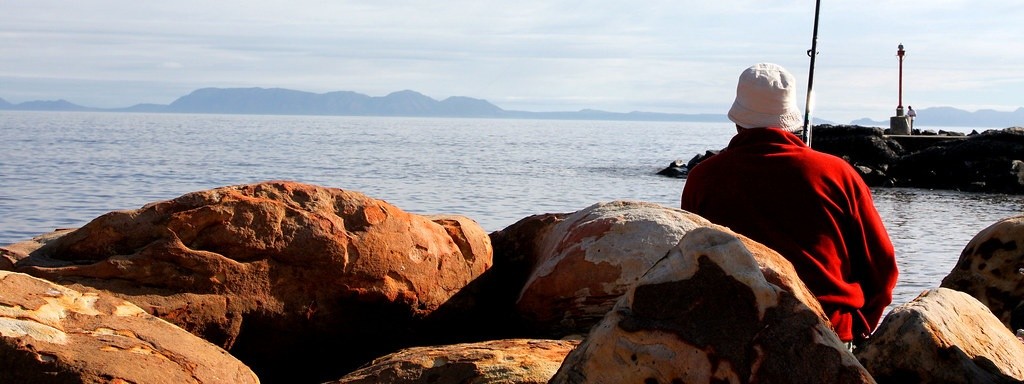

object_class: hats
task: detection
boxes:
[728,63,805,131]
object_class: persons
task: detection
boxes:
[680,62,899,353]
[908,106,916,134]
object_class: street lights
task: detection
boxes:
[896,42,906,116]
